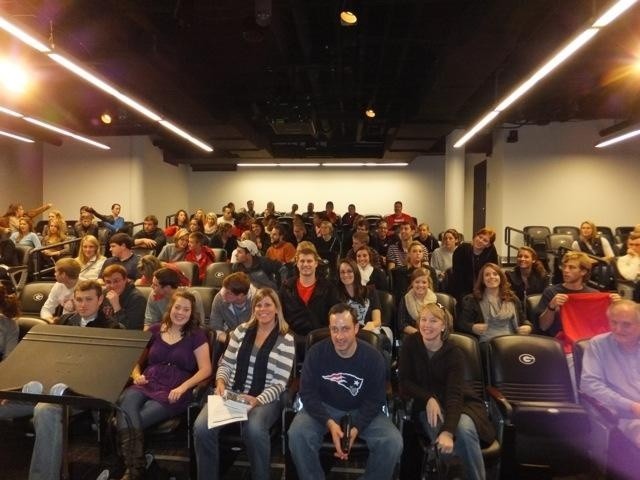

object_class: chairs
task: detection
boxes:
[0,219,133,478]
[500,225,640,333]
[479,333,589,480]
[397,332,506,480]
[193,331,296,479]
[105,330,220,479]
[291,328,394,479]
[573,337,638,480]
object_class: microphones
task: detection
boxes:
[50,383,116,410]
[5,381,43,394]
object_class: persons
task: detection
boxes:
[0,279,128,480]
[399,303,497,480]
[580,300,640,449]
[192,288,297,480]
[286,303,404,480]
[100,291,213,480]
[0,200,640,364]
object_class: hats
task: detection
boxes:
[237,239,258,256]
[174,229,189,240]
